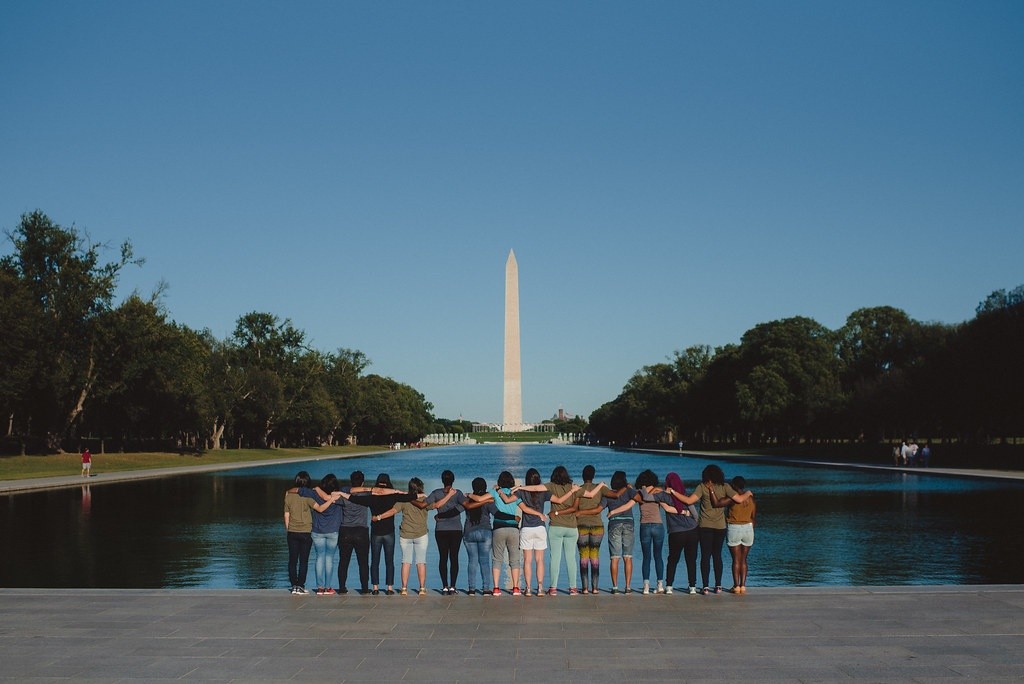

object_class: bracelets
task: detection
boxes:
[395,489,397,494]
[670,489,675,493]
[377,515,383,520]
[711,491,715,494]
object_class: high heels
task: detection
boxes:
[442,586,460,597]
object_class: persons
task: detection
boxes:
[284,465,755,597]
[679,441,683,451]
[389,441,429,451]
[81,448,92,478]
[892,441,932,468]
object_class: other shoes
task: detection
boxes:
[339,586,369,594]
[592,588,600,594]
[582,587,589,594]
[373,589,394,595]
[317,587,336,595]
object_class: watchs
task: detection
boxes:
[554,511,558,515]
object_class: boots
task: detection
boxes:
[657,581,664,594]
[642,579,650,594]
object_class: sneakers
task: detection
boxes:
[568,587,579,595]
[524,587,533,596]
[512,586,521,595]
[493,587,502,595]
[687,584,696,595]
[536,588,546,597]
[701,587,710,595]
[611,588,620,595]
[625,588,633,595]
[401,587,408,595]
[467,590,475,596]
[291,586,308,595]
[740,585,746,594]
[483,590,493,596]
[665,586,674,594]
[729,585,741,594]
[714,586,722,594]
[419,587,427,594]
[545,588,557,596]
